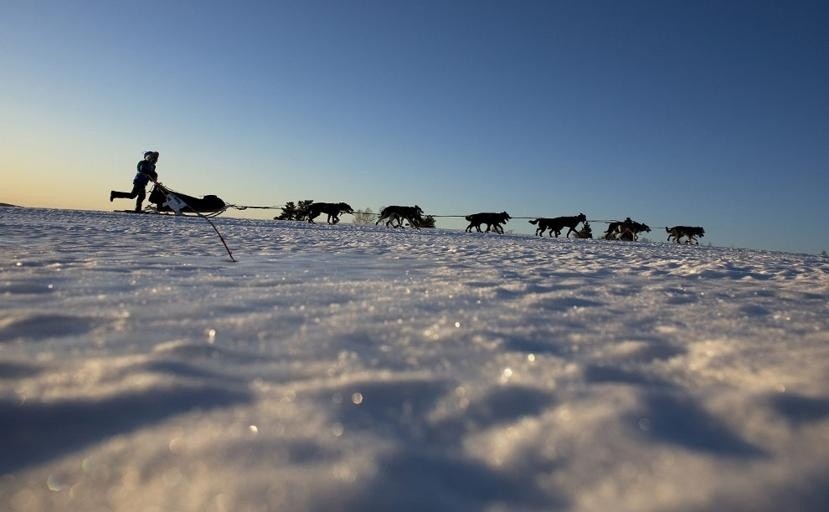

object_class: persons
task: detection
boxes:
[109,150,161,213]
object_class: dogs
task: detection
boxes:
[547,211,588,238]
[602,221,651,242]
[464,211,512,233]
[664,224,706,245]
[305,201,354,225]
[527,217,561,238]
[375,205,425,228]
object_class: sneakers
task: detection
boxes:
[111,191,115,201]
[135,207,142,211]
